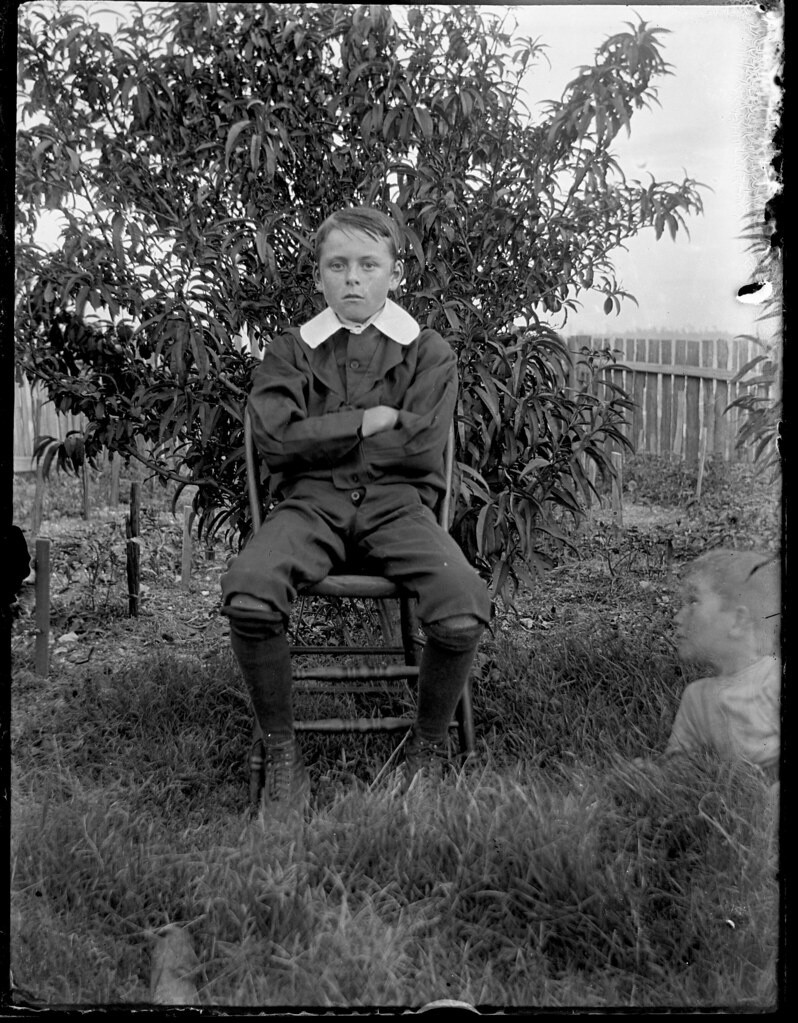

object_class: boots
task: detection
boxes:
[403,724,450,803]
[256,737,311,826]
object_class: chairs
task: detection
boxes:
[240,402,478,795]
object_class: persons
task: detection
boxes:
[214,207,493,836]
